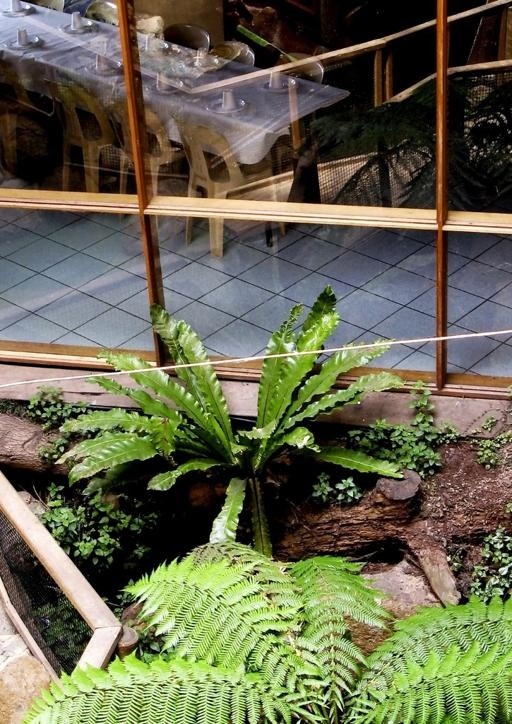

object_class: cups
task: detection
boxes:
[9,0,283,110]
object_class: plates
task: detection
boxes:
[0,3,298,116]
